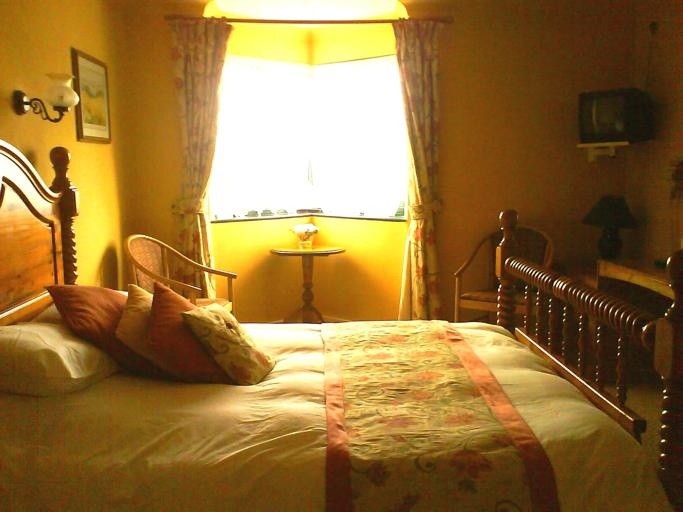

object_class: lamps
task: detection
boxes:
[12,72,79,123]
[580,194,637,259]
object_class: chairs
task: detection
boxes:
[453,224,553,327]
[123,234,237,321]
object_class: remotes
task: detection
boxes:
[655,260,666,267]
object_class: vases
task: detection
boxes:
[299,240,311,249]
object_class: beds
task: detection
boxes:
[0,138,681,512]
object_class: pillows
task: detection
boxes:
[177,303,278,388]
[115,284,182,377]
[143,281,232,383]
[43,284,151,372]
[30,291,128,328]
[0,320,116,397]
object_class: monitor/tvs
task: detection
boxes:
[577,87,653,144]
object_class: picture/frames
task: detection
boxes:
[70,47,110,145]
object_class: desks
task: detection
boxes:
[562,258,682,354]
[268,248,345,323]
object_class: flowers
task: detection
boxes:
[292,224,318,242]
